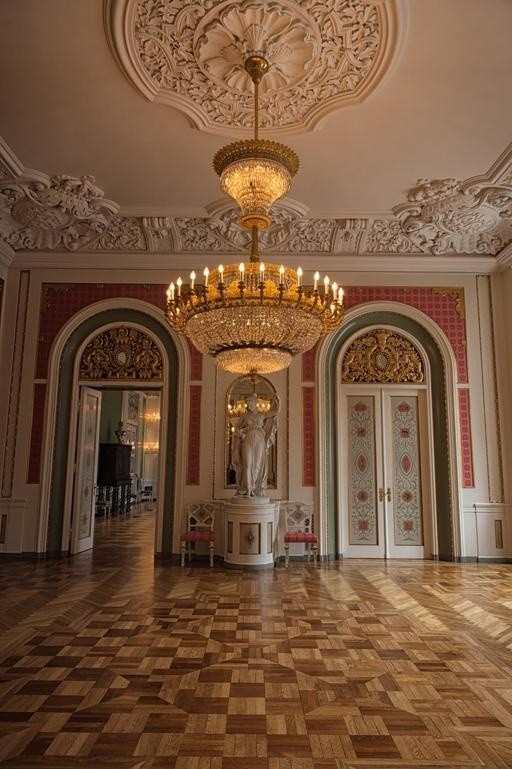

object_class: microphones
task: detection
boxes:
[95,501,111,520]
[137,491,154,513]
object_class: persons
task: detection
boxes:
[235,391,282,496]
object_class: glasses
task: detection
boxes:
[165,56,345,384]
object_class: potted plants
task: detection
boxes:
[120,390,140,427]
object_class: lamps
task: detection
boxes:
[283,502,319,567]
[178,500,217,568]
